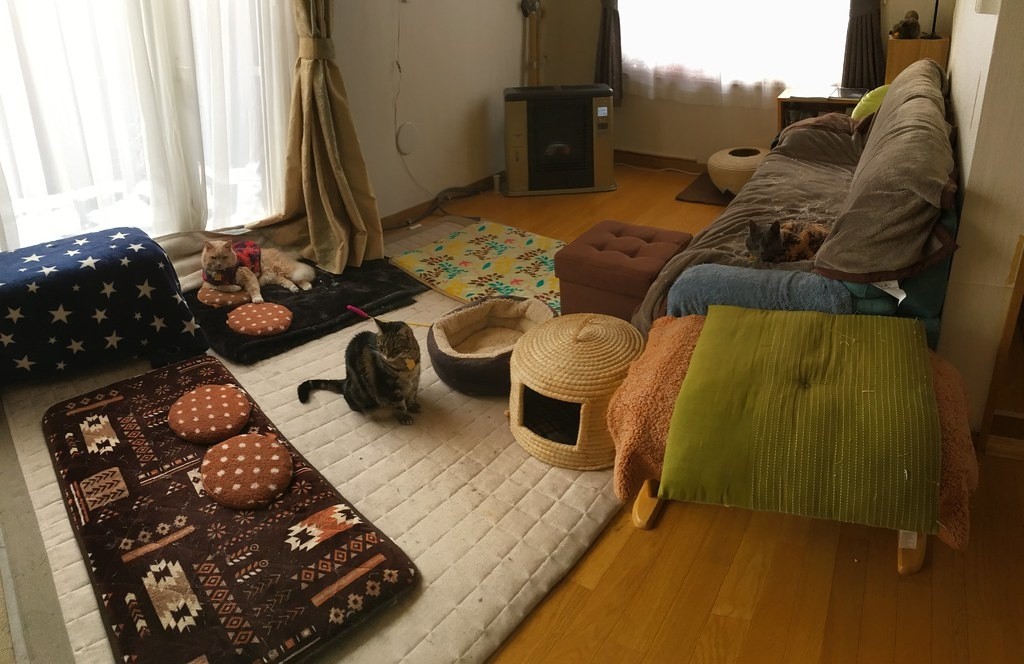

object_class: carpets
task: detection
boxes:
[675,171,736,207]
[394,217,568,318]
[181,255,430,365]
[0,210,629,664]
[35,356,427,664]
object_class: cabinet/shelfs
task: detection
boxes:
[774,85,872,132]
[884,33,949,85]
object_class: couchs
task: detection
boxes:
[635,54,965,349]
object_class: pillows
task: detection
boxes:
[655,304,939,537]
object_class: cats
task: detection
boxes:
[745,219,830,262]
[201,240,318,304]
[297,316,422,426]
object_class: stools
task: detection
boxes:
[608,303,979,574]
[0,226,208,392]
[553,219,693,321]
